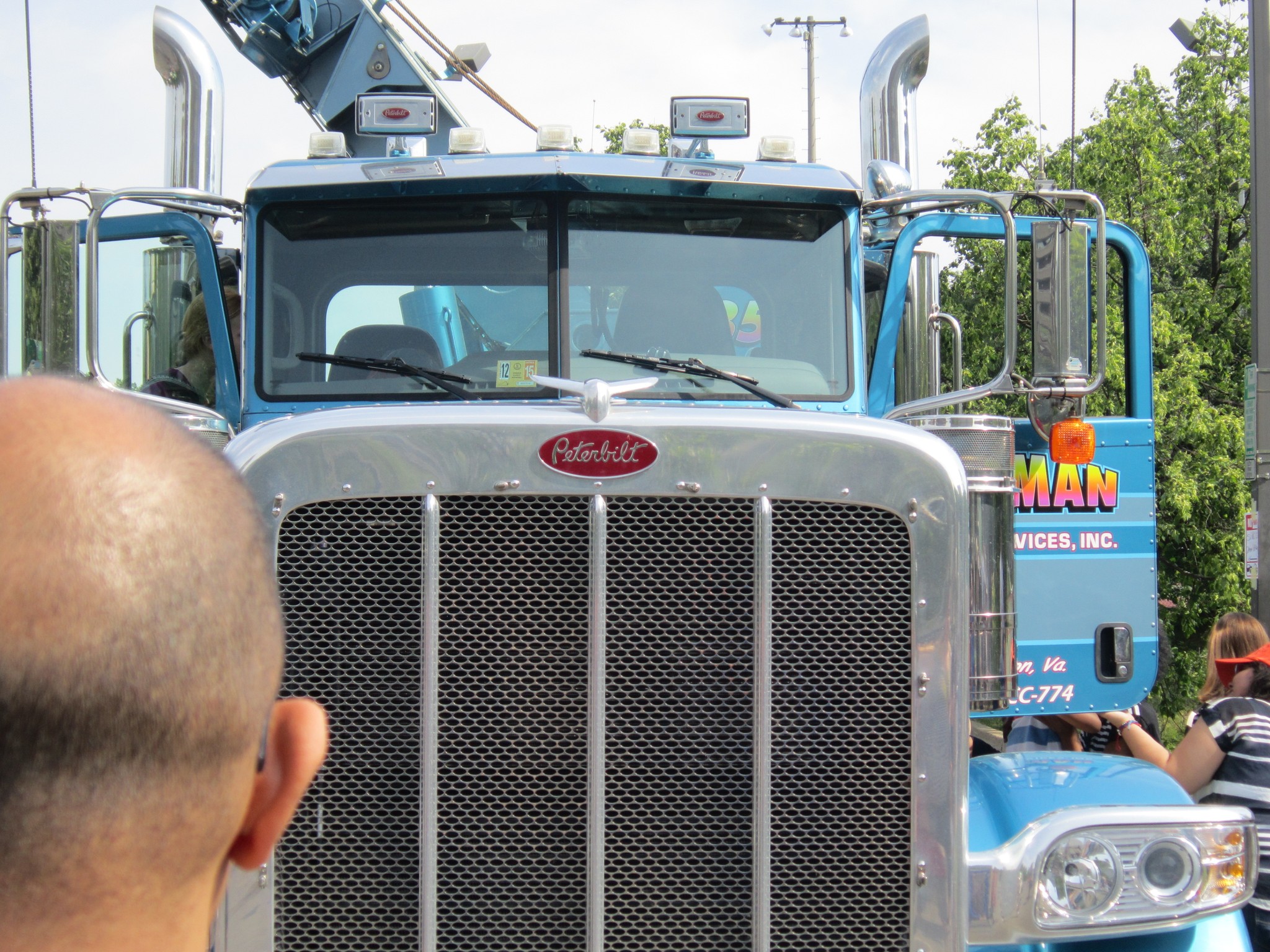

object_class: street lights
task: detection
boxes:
[1168,17,1247,255]
[763,17,851,164]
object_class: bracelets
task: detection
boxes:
[1117,720,1142,736]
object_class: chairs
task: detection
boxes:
[326,324,444,382]
[610,283,737,356]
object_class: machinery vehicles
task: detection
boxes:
[1,2,1262,952]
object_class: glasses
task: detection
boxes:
[1235,663,1259,673]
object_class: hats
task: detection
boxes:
[1215,643,1270,688]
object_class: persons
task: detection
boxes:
[0,377,328,952]
[1001,612,1270,952]
[140,287,241,408]
